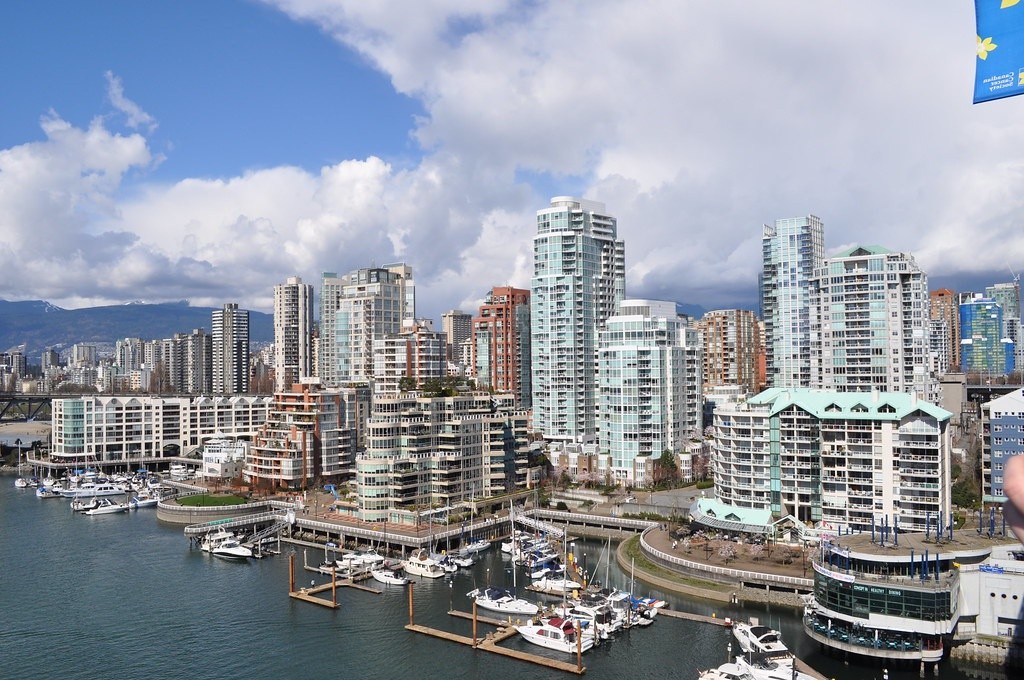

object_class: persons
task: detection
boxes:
[659,523,667,529]
[303,507,309,515]
[311,579,315,588]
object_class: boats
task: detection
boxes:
[14,435,176,516]
[426,498,474,567]
[319,560,347,573]
[326,557,363,566]
[342,546,384,564]
[697,653,818,680]
[400,550,446,579]
[465,586,539,615]
[199,528,245,552]
[212,540,252,559]
[732,621,788,656]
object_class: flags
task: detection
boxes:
[824,521,833,529]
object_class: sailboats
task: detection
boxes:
[466,483,491,554]
[501,498,670,655]
[369,516,409,585]
[424,500,457,572]
